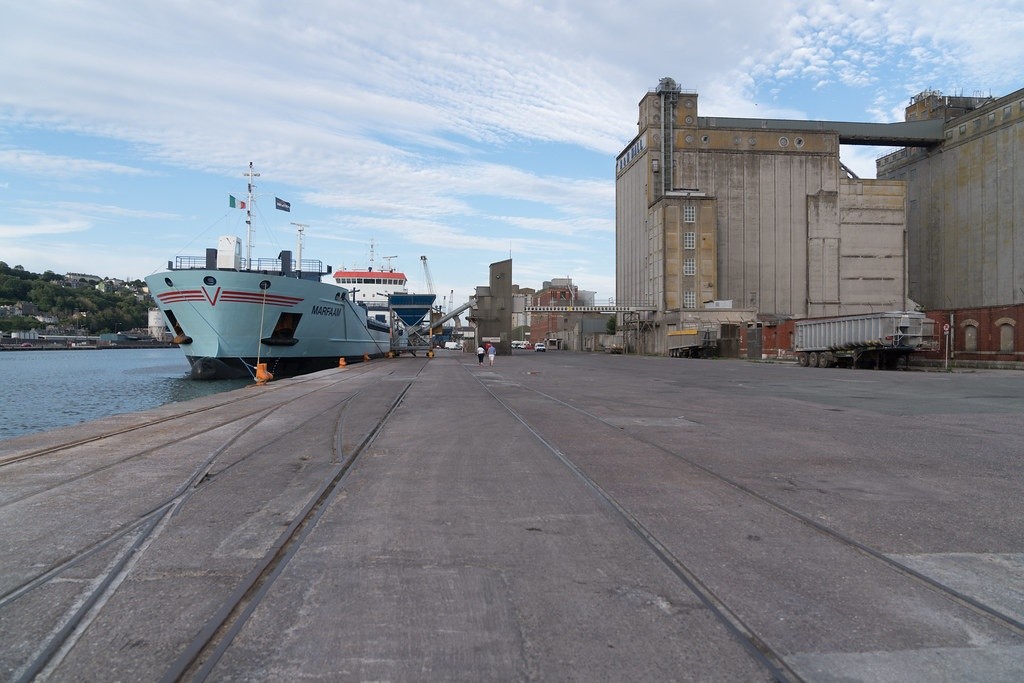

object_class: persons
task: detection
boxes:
[487,344,496,366]
[477,345,486,365]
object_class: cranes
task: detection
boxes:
[419,255,455,327]
[339,239,398,270]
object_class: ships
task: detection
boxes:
[144,160,390,381]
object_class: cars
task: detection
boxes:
[534,343,547,352]
[511,341,532,349]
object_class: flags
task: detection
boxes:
[276,198,290,212]
[230,196,245,209]
